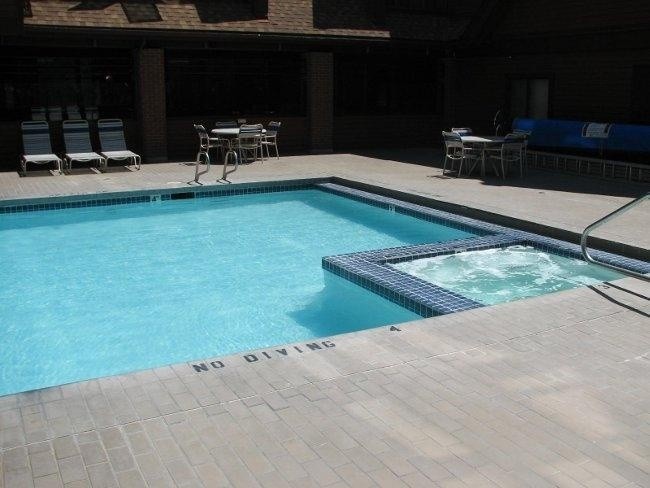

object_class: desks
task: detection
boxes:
[211,126,254,160]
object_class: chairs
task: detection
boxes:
[194,120,234,163]
[216,119,238,155]
[59,119,106,174]
[16,116,65,178]
[96,117,142,173]
[229,124,264,162]
[260,121,283,161]
[441,122,534,182]
